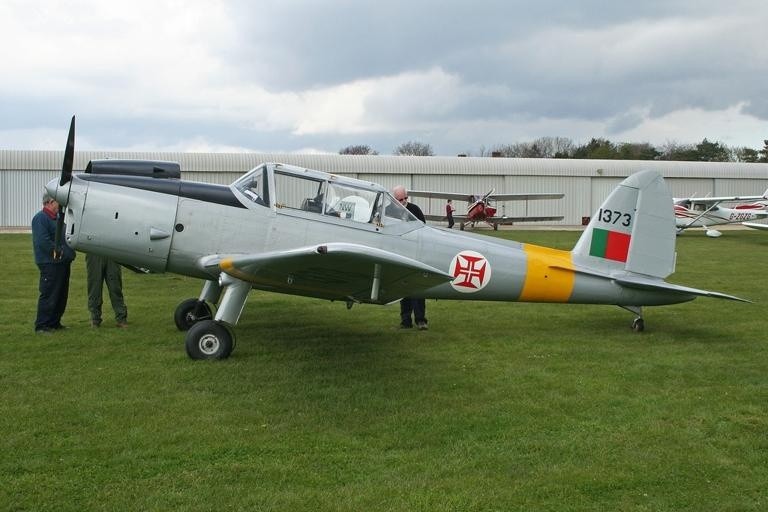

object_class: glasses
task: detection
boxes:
[398,196,409,201]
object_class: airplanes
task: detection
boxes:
[402,186,565,234]
[42,113,754,361]
[670,188,768,238]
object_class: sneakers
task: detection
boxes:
[417,320,428,331]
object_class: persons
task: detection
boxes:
[384,187,432,330]
[29,193,76,334]
[83,250,130,329]
[446,199,456,229]
